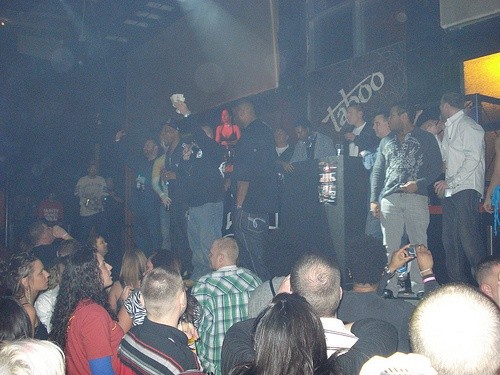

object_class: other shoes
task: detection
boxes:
[417,292,424,300]
[383,289,392,299]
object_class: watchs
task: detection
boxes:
[183,113,190,118]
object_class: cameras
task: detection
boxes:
[408,244,418,257]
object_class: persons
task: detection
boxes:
[0,223,500,375]
[38,193,64,226]
[215,109,334,174]
[343,102,374,156]
[483,137,500,256]
[75,160,112,259]
[114,131,162,266]
[357,102,443,298]
[152,116,193,279]
[289,118,336,164]
[161,100,225,283]
[373,99,447,285]
[231,99,279,282]
[434,92,487,284]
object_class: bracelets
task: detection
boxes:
[235,205,242,208]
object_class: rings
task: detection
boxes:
[166,175,167,177]
